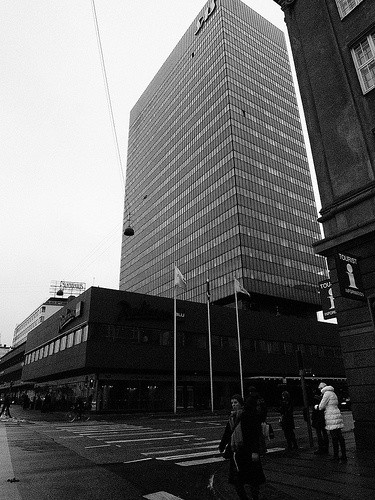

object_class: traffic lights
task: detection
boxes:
[90,379,94,388]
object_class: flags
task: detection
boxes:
[174,266,187,289]
[206,276,210,302]
[234,279,250,296]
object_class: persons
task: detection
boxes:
[218,394,268,500]
[0,391,94,420]
[246,385,269,457]
[279,391,298,451]
[308,382,348,462]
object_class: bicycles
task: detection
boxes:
[65,403,91,423]
